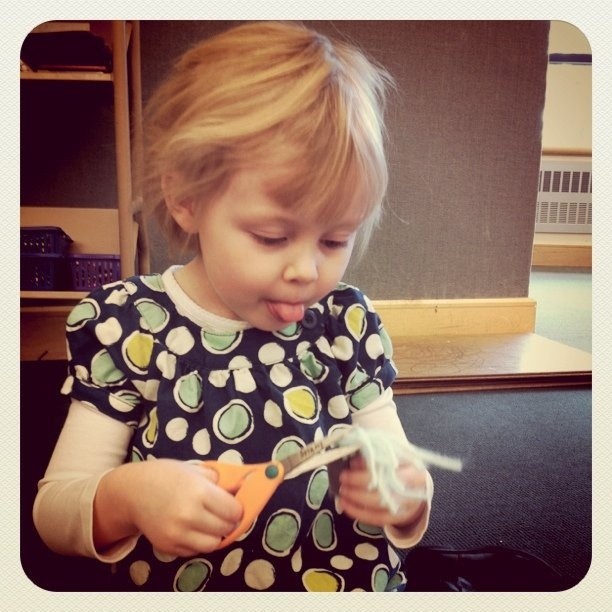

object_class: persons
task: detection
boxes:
[31,21,434,592]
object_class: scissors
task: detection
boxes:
[199,423,363,550]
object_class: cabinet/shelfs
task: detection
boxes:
[18,22,151,364]
